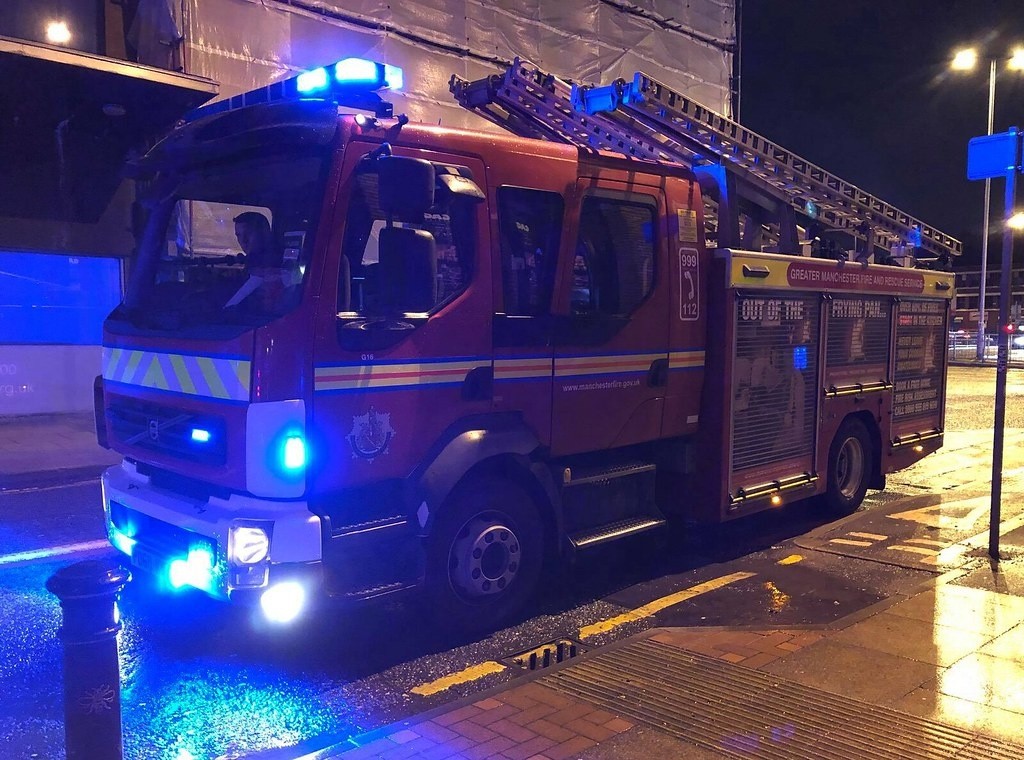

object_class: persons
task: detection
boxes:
[232,211,274,272]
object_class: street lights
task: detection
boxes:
[952,49,1024,361]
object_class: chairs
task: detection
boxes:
[449,200,532,314]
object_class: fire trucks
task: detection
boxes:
[89,59,961,633]
